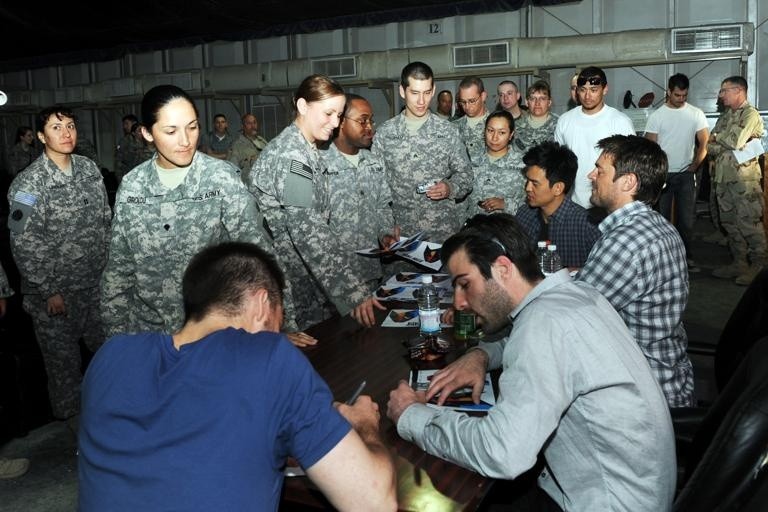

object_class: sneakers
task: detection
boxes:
[686,261,702,278]
[0,455,31,484]
[711,259,763,286]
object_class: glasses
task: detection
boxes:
[576,74,605,91]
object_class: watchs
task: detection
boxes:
[713,132,717,139]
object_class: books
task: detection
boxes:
[733,139,764,165]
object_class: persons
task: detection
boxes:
[385,214,679,512]
[707,75,768,284]
[640,74,711,273]
[552,66,637,224]
[704,106,730,245]
[515,142,600,271]
[567,134,697,413]
[568,74,581,110]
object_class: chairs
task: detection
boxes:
[667,345,766,510]
[670,262,766,467]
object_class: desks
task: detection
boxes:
[278,291,515,512]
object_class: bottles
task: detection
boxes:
[417,274,444,363]
[541,241,551,262]
[542,244,561,273]
[534,240,551,273]
[452,305,480,341]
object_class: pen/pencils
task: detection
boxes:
[409,368,413,387]
[348,380,367,406]
